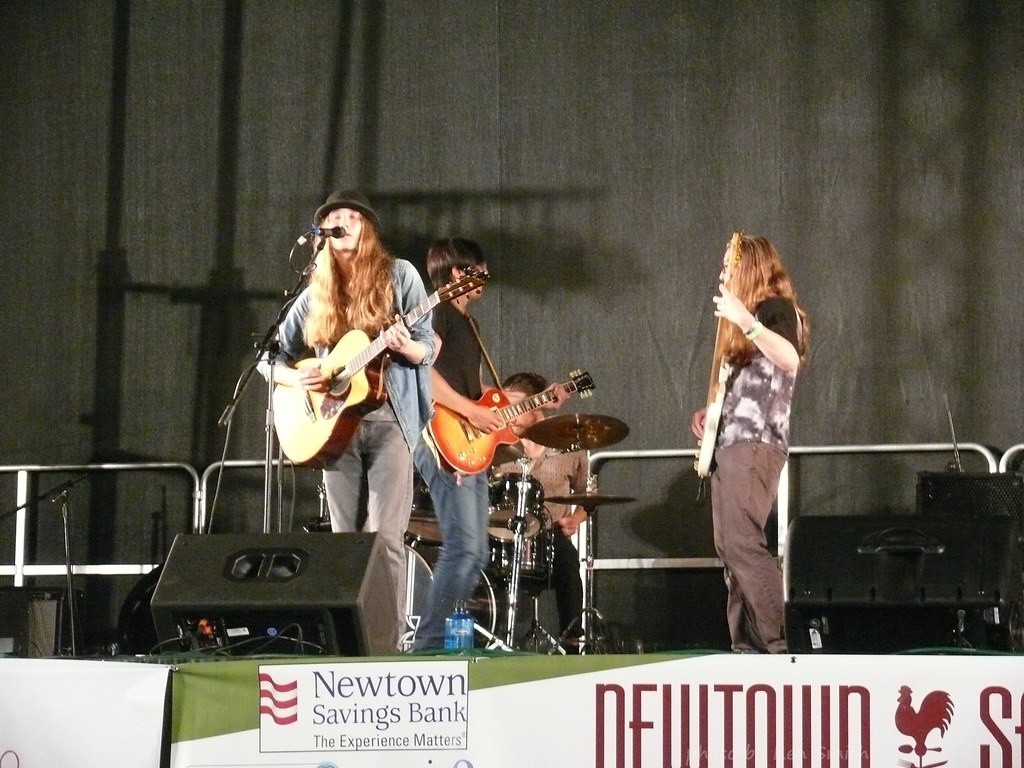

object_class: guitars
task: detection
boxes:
[427,369,595,480]
[694,229,747,477]
[271,264,490,469]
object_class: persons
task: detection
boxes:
[256,190,441,657]
[494,372,591,650]
[694,234,811,654]
[413,236,570,651]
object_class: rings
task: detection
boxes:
[394,345,401,351]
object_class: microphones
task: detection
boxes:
[311,227,346,239]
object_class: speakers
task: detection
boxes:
[150,531,399,658]
[915,472,1024,515]
[783,515,1024,656]
[0,586,87,660]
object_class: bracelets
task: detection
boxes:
[743,318,763,341]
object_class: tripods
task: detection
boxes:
[519,512,626,657]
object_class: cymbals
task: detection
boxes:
[544,493,636,506]
[525,411,630,451]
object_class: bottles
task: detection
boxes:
[444,600,474,650]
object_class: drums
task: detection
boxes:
[487,472,545,540]
[400,540,496,651]
[405,471,446,542]
[318,474,336,529]
[490,528,555,582]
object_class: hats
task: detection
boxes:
[313,189,381,230]
